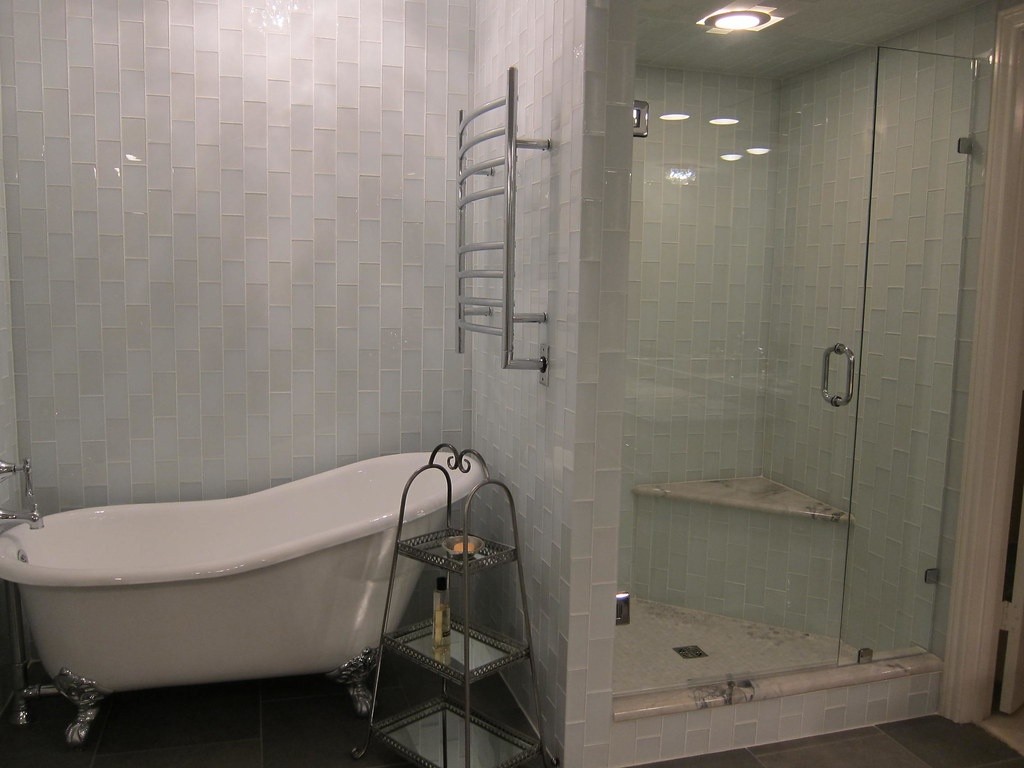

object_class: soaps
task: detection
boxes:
[453,540,476,554]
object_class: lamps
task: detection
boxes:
[696,0,784,35]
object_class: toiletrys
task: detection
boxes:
[432,576,453,648]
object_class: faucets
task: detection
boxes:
[0,503,44,530]
[0,457,36,497]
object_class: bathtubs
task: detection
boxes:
[0,451,488,702]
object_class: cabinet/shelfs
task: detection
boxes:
[349,442,560,768]
